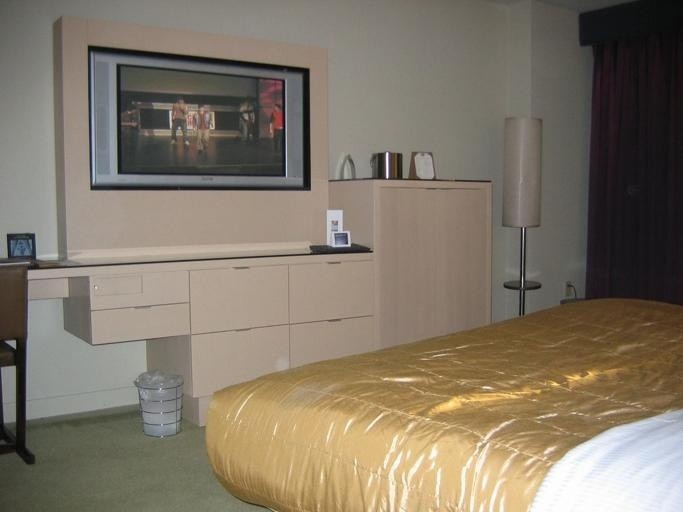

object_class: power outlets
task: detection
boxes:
[563,281,574,297]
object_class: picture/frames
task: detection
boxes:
[7,233,37,261]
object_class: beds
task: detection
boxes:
[207,300,683,512]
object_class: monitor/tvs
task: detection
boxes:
[86,44,311,191]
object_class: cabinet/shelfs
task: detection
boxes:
[27,252,374,427]
[330,181,492,351]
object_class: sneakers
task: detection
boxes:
[185,140,189,144]
[171,140,174,142]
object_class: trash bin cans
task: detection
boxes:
[133,372,185,437]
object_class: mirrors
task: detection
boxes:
[408,152,435,180]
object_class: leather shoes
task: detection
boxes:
[203,145,206,149]
[198,149,201,152]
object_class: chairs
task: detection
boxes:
[0,264,37,465]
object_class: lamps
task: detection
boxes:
[502,117,542,316]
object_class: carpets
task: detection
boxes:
[0,407,267,512]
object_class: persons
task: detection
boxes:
[169,95,190,146]
[193,103,211,150]
[268,104,283,151]
[238,97,255,140]
